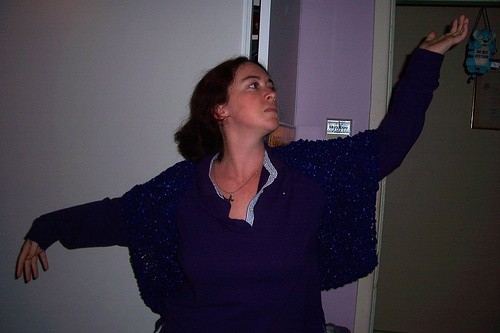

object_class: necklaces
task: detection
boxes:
[212,160,264,202]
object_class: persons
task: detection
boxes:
[15,15,470,332]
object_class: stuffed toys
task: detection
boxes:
[466,28,498,80]
